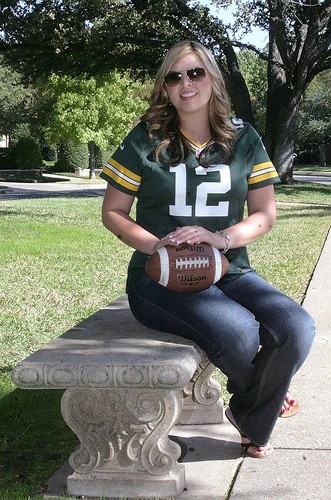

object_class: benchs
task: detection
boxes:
[10,294,223,498]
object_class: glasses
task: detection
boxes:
[164,67,208,87]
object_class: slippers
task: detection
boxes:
[225,407,274,458]
[278,390,300,418]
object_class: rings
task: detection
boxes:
[195,230,199,237]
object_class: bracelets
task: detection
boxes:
[217,230,231,255]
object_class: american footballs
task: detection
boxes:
[145,242,230,293]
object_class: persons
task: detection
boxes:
[98,38,315,457]
